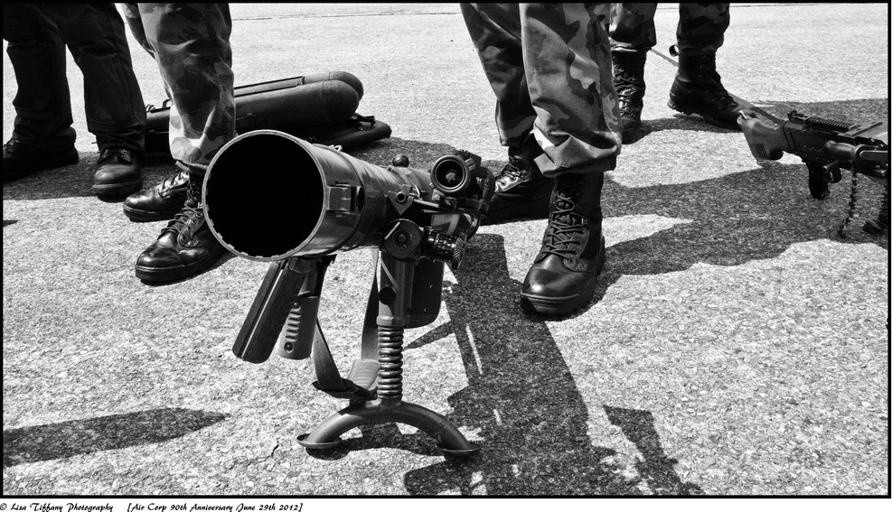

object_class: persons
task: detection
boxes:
[119,0,246,284]
[609,0,761,146]
[455,1,627,323]
[0,0,149,202]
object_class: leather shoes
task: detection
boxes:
[91,129,149,201]
[2,118,82,184]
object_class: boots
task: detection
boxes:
[607,50,648,148]
[124,171,197,222]
[667,48,762,137]
[515,172,607,324]
[134,192,227,287]
[475,136,555,225]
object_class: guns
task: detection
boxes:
[202,129,495,457]
[737,107,890,242]
